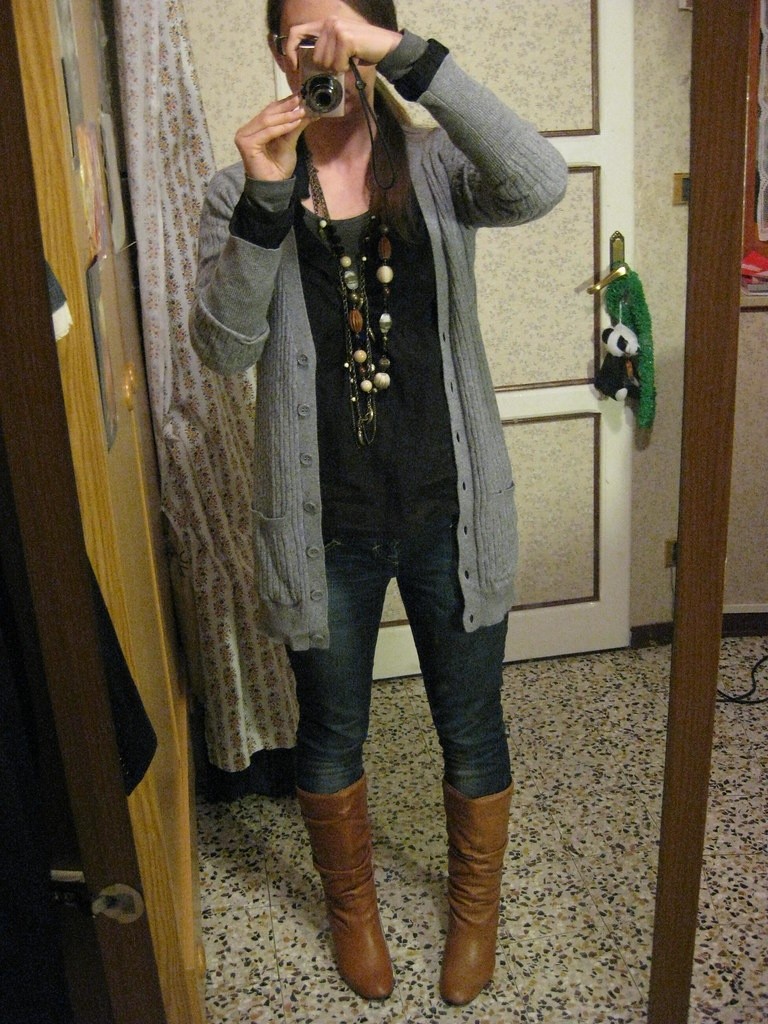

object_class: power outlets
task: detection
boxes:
[665,538,676,570]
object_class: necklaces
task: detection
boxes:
[305,150,394,450]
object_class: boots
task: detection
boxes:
[295,769,394,1000]
[440,776,514,1006]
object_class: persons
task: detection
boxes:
[189,0,568,1007]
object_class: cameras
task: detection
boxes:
[297,45,346,118]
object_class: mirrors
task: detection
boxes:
[0,0,755,1024]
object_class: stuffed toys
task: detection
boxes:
[594,323,644,400]
